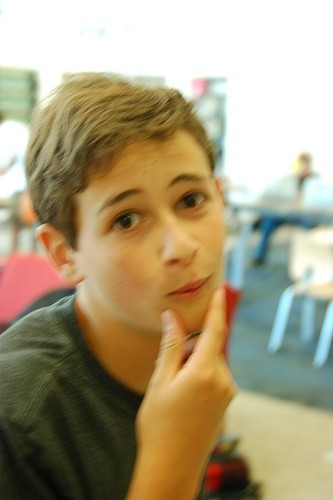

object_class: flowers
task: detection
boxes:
[204,437,253,497]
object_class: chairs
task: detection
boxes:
[270,226,333,367]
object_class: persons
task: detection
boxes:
[244,152,321,275]
[0,69,263,500]
